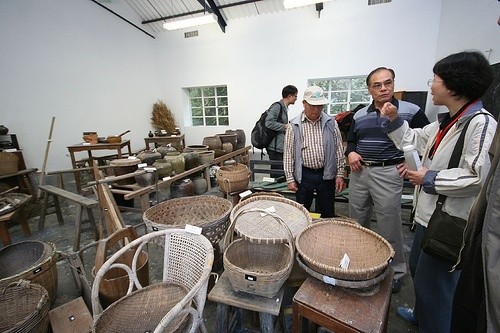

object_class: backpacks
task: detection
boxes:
[250,102,283,161]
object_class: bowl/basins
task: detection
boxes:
[172,178,196,195]
[107,135,122,143]
[186,144,214,164]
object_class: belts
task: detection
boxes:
[361,160,406,167]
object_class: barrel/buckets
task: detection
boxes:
[0,240,65,305]
[0,148,19,175]
[216,133,238,154]
[92,225,149,305]
[225,129,246,148]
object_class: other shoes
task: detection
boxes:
[391,279,401,294]
[396,306,417,327]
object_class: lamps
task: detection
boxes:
[163,0,218,30]
[283,0,330,10]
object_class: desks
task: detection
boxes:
[0,168,38,205]
[144,133,185,151]
[67,140,132,194]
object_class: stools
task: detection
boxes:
[208,271,285,333]
[293,267,395,333]
[49,297,93,333]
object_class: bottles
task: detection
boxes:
[403,145,423,172]
[83,132,98,143]
[111,145,185,188]
[193,176,207,194]
[0,125,8,135]
[181,149,200,171]
[203,136,221,157]
[148,128,180,137]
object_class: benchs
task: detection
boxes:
[39,185,100,252]
[250,160,417,232]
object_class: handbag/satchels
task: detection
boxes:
[421,207,467,265]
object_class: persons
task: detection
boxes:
[345,67,432,294]
[267,85,298,178]
[381,51,497,333]
[283,86,346,218]
[339,104,365,140]
[451,118,500,333]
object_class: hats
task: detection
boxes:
[302,86,329,107]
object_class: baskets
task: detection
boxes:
[221,207,296,300]
[230,195,313,247]
[144,194,233,257]
[215,164,252,194]
[93,281,194,333]
[0,280,52,333]
[295,217,396,297]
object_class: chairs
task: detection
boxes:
[91,229,214,333]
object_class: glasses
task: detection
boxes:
[427,77,442,87]
